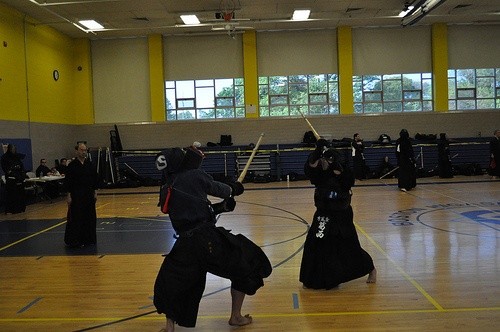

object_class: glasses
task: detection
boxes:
[42,162,46,163]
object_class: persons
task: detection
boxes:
[395,129,417,192]
[153,148,272,332]
[299,139,376,289]
[438,133,454,178]
[351,133,369,181]
[488,130,500,176]
[36,157,68,200]
[65,140,98,255]
[0,144,27,216]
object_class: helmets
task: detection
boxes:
[400,129,409,138]
[165,148,186,173]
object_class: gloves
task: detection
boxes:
[220,197,236,212]
[227,182,244,197]
[308,139,327,164]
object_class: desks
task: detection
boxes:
[26,175,65,204]
